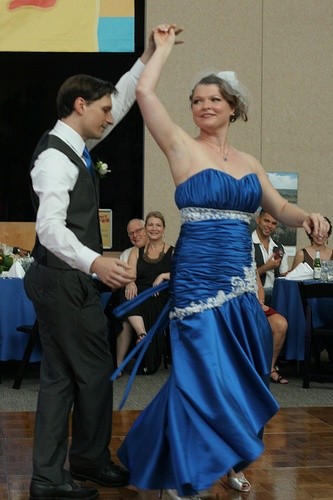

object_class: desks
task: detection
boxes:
[0,277,113,362]
[272,277,333,388]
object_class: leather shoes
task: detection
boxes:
[30,482,100,500]
[69,459,132,487]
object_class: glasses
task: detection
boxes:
[128,227,145,237]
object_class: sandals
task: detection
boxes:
[271,371,287,384]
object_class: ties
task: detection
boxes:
[82,146,93,181]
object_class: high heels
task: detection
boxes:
[158,485,202,500]
[227,469,250,492]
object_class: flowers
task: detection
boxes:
[96,160,111,176]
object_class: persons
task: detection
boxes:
[103,218,332,385]
[115,26,330,500]
[23,26,183,500]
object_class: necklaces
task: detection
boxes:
[209,147,230,162]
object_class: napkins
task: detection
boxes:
[283,262,314,280]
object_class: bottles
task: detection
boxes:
[321,261,329,283]
[313,249,321,280]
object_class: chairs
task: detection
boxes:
[12,278,115,389]
